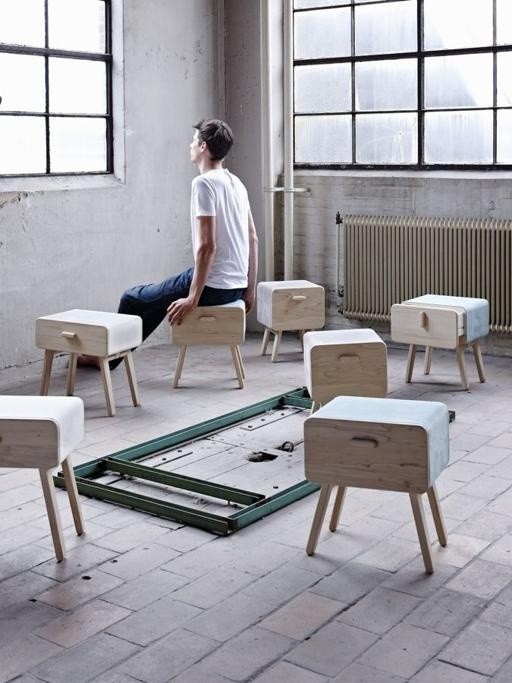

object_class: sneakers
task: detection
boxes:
[67,352,103,371]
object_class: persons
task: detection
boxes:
[69,119,260,371]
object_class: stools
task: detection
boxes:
[171,299,247,389]
[391,295,489,392]
[0,393,85,561]
[35,309,142,416]
[304,395,449,575]
[256,279,325,362]
[303,328,387,416]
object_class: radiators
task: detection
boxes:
[335,210,511,335]
